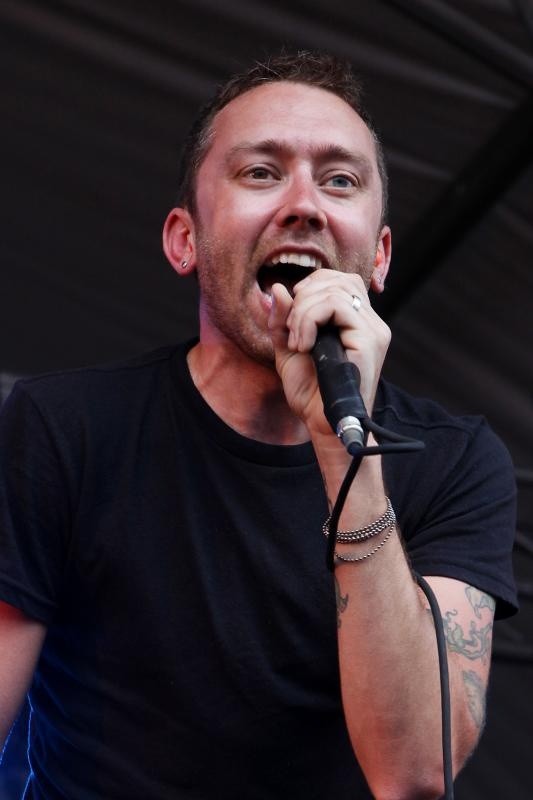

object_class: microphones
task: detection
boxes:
[308,325,369,458]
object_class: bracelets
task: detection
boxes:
[322,494,396,563]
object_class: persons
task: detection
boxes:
[0,47,520,800]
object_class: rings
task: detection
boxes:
[352,293,362,310]
